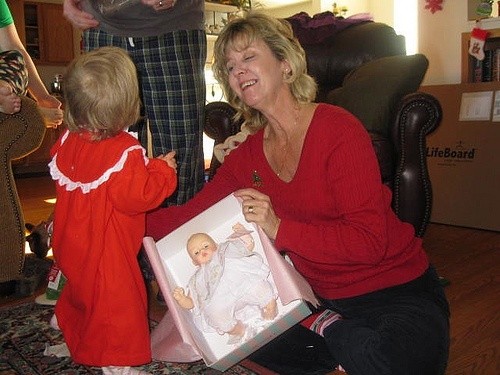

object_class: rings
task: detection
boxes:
[159,1,162,7]
[249,207,253,213]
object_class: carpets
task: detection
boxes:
[0,299,254,375]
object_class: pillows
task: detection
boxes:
[328,47,428,133]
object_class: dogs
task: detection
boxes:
[24,206,54,259]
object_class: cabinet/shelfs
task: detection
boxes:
[204,0,253,66]
[17,94,65,173]
[6,0,77,64]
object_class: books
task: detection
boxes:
[473,49,500,82]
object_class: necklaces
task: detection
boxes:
[268,121,297,178]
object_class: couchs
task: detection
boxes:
[203,16,441,242]
[0,97,47,290]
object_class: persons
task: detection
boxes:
[62,0,207,305]
[173,222,279,343]
[145,11,450,375]
[0,0,64,129]
[48,46,178,367]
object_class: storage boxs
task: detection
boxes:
[420,83,500,232]
